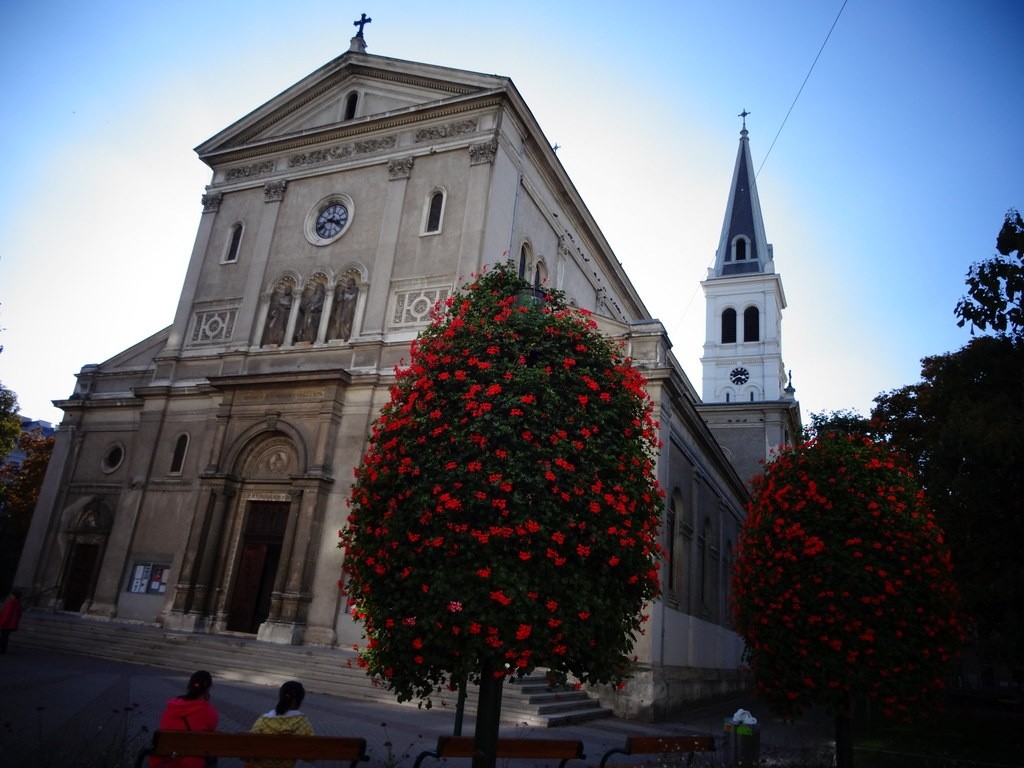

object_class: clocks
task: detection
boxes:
[315,203,349,239]
[729,368,750,386]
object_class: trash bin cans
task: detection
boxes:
[723,718,761,768]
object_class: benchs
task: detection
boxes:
[600,735,715,768]
[133,729,370,768]
[413,735,587,768]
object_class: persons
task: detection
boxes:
[0,590,22,654]
[147,670,220,768]
[244,681,315,768]
[265,287,292,345]
[327,279,359,341]
[295,283,325,344]
